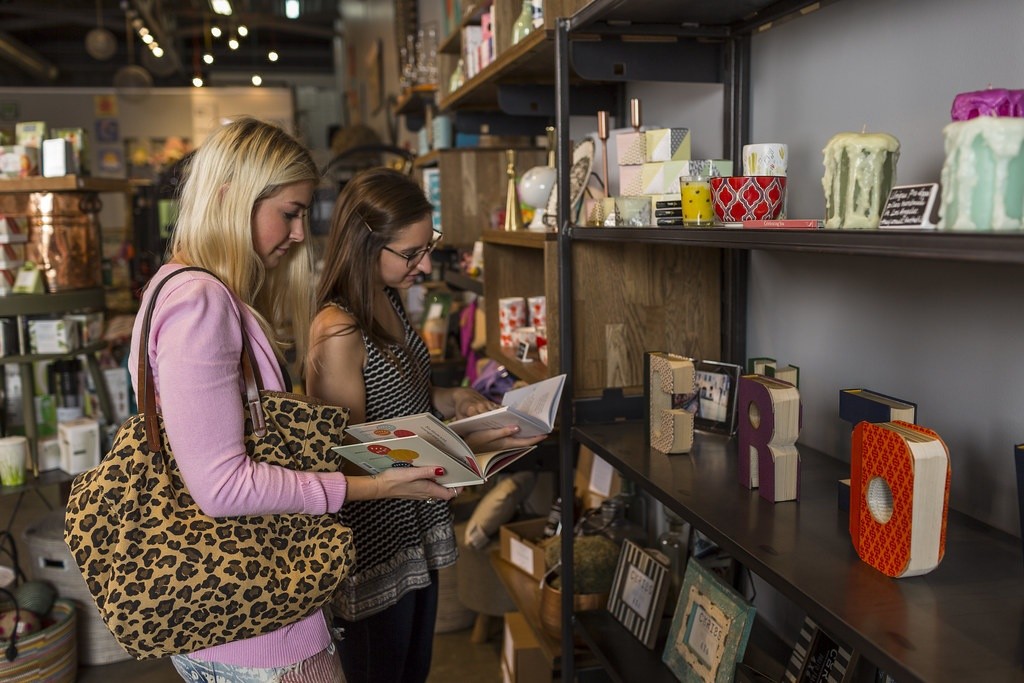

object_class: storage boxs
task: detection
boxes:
[498,516,554,584]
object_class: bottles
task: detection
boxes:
[511,0,535,46]
[448,61,465,94]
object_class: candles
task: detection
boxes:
[821,120,900,230]
[936,84,1024,235]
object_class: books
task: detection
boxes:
[448,374,567,438]
[330,413,537,488]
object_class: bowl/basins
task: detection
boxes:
[538,572,609,650]
[709,176,786,221]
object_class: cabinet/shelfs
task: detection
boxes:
[0,174,133,446]
[393,0,1024,682]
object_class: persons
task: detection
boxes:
[303,168,547,683]
[130,117,465,683]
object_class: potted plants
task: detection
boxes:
[538,533,619,647]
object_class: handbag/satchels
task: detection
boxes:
[0,531,82,683]
[61,265,360,664]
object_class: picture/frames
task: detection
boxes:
[606,538,666,651]
[691,360,739,439]
[662,554,755,683]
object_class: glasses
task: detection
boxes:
[382,227,444,269]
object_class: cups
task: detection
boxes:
[680,175,716,227]
[742,144,788,221]
[498,295,548,366]
[0,436,27,485]
[431,116,454,150]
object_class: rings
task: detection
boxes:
[425,496,437,504]
[453,488,458,498]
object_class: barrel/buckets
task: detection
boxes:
[0,192,104,297]
